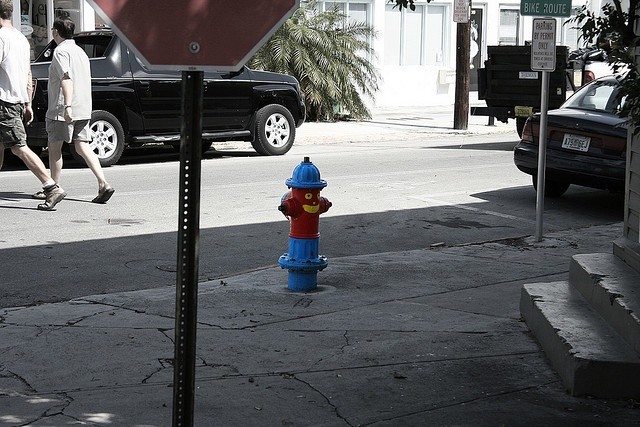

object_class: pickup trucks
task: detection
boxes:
[0,29,306,171]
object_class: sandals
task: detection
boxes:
[31,192,46,199]
[92,184,115,203]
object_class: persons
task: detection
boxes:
[0,0,66,210]
[45,16,115,203]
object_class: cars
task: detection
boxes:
[514,73,628,198]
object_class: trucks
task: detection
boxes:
[477,43,628,139]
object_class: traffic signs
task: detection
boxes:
[520,0,571,17]
[453,0,470,23]
[531,18,557,72]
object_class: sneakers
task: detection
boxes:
[38,182,67,210]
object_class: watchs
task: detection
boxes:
[63,105,71,109]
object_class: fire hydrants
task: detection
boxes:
[278,157,333,293]
